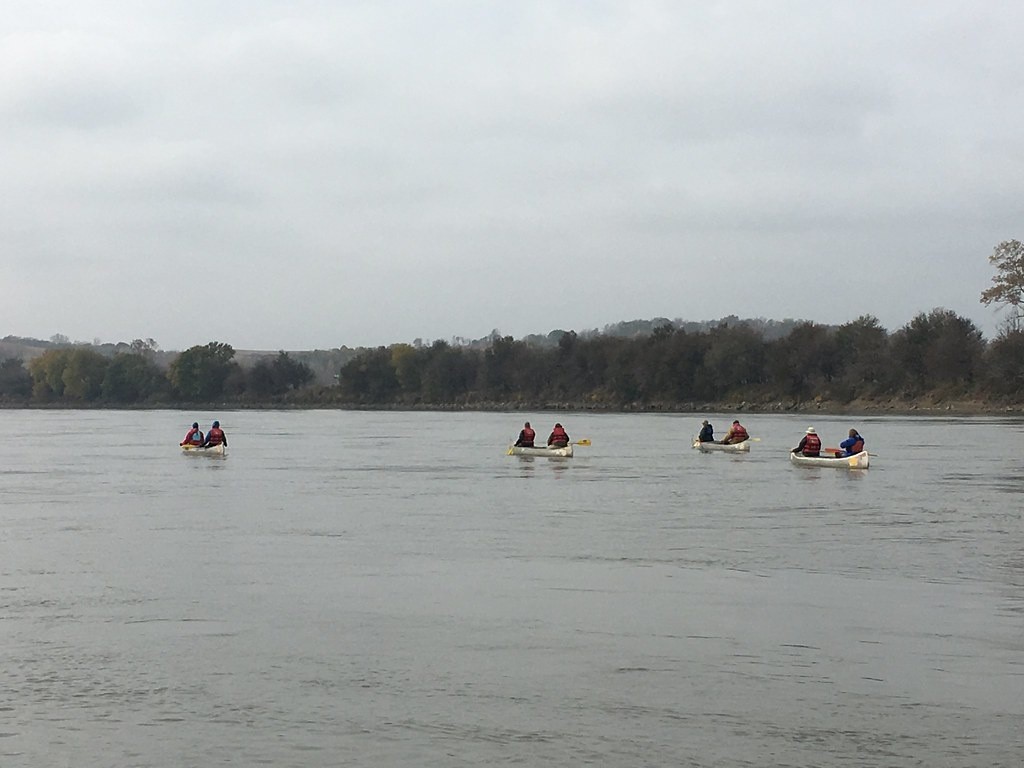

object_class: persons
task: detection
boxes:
[698,420,714,442]
[197,420,227,449]
[180,422,204,446]
[513,422,535,447]
[548,423,569,446]
[720,420,749,444]
[790,426,821,458]
[835,428,864,458]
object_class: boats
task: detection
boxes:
[791,451,868,470]
[694,440,750,453]
[508,445,574,459]
[181,444,225,454]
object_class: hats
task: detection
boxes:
[214,421,219,426]
[806,427,816,434]
[192,422,197,427]
[702,420,708,424]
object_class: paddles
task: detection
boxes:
[568,440,592,446]
[749,436,760,442]
[506,446,515,455]
[826,447,878,457]
